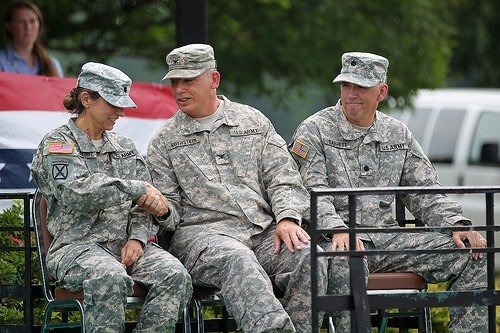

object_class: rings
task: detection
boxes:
[296,231,301,235]
[479,238,484,241]
[136,257,139,259]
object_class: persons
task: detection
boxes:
[0,0,65,79]
[28,43,327,333]
[286,52,490,333]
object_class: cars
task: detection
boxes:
[380,88,500,274]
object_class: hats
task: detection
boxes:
[77,62,137,108]
[161,44,215,82]
[333,52,389,88]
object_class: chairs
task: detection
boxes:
[30,186,461,333]
